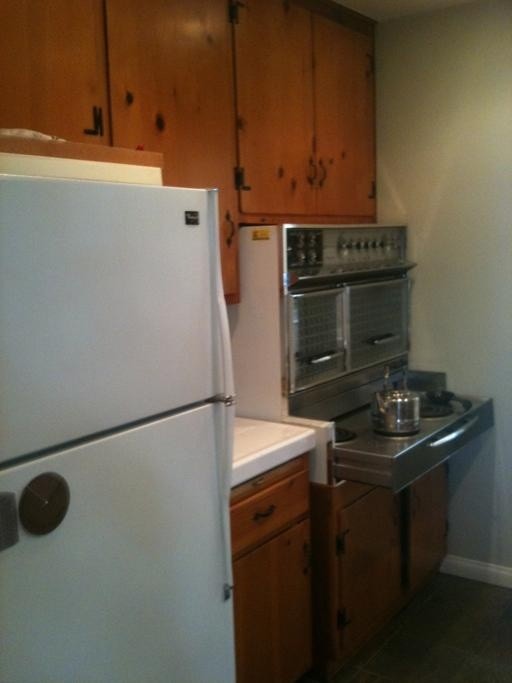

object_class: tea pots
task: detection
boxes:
[371,360,420,433]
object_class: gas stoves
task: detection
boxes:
[328,398,453,447]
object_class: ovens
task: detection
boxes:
[282,277,414,393]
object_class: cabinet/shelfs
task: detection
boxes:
[0,0,112,154]
[321,459,454,679]
[227,459,313,683]
[105,1,241,307]
[232,1,379,226]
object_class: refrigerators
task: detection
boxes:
[0,175,237,683]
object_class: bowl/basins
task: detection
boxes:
[427,390,453,403]
